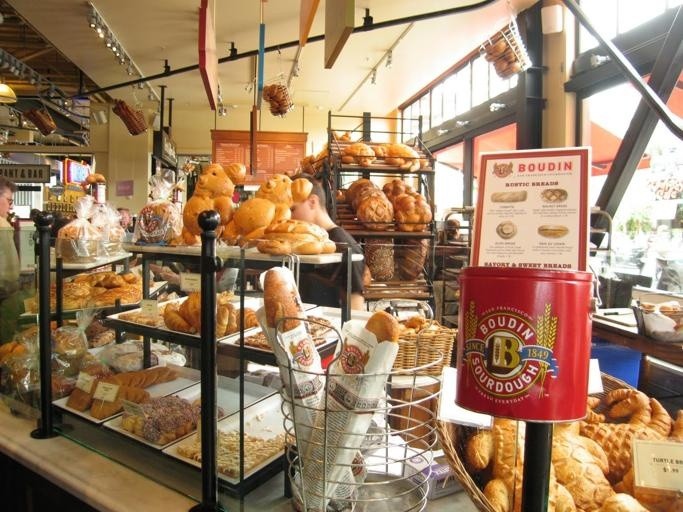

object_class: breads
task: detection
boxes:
[235,331,272,353]
[221,174,313,249]
[122,396,224,446]
[397,238,428,281]
[364,237,395,281]
[264,267,400,512]
[257,220,337,257]
[497,220,516,241]
[374,144,420,173]
[490,190,527,203]
[0,316,158,409]
[465,388,683,511]
[542,189,567,203]
[177,431,296,481]
[384,180,432,232]
[538,224,568,237]
[138,204,179,245]
[182,163,246,246]
[28,273,155,314]
[639,302,683,317]
[398,317,453,334]
[340,143,375,169]
[346,178,393,231]
[304,315,333,346]
[163,292,258,336]
[56,227,123,265]
[119,301,180,327]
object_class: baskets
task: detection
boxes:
[480,17,533,79]
[435,369,637,512]
[391,318,457,375]
[275,317,441,512]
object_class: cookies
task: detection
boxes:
[66,366,177,419]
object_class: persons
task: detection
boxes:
[289,172,366,310]
[435,219,469,282]
[0,175,25,349]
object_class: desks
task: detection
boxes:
[0,391,482,512]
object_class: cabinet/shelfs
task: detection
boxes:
[442,204,613,329]
[323,109,437,309]
[210,129,308,176]
[18,205,364,511]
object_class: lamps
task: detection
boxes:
[455,120,471,127]
[87,8,159,115]
[385,48,393,67]
[490,102,507,113]
[370,66,378,85]
[0,83,18,104]
[590,54,612,67]
[437,128,449,137]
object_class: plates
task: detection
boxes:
[155,296,318,341]
[109,291,254,329]
[50,360,202,424]
[161,388,300,485]
[106,375,279,453]
[216,305,375,357]
